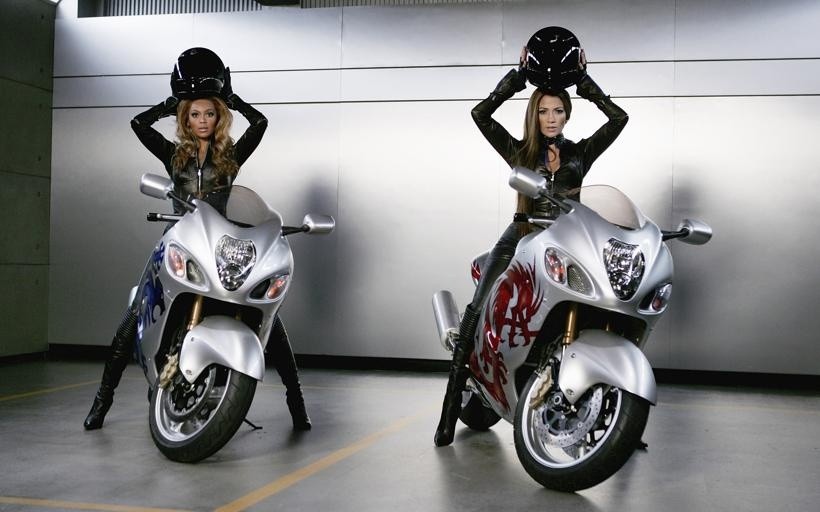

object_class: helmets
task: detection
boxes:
[171,48,225,100]
[524,27,581,88]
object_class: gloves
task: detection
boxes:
[220,67,233,98]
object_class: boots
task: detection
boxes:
[266,318,312,430]
[434,304,480,446]
[84,308,138,429]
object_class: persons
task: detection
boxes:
[432,42,652,454]
[79,67,314,433]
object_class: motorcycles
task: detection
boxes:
[126,170,336,465]
[430,165,714,493]
